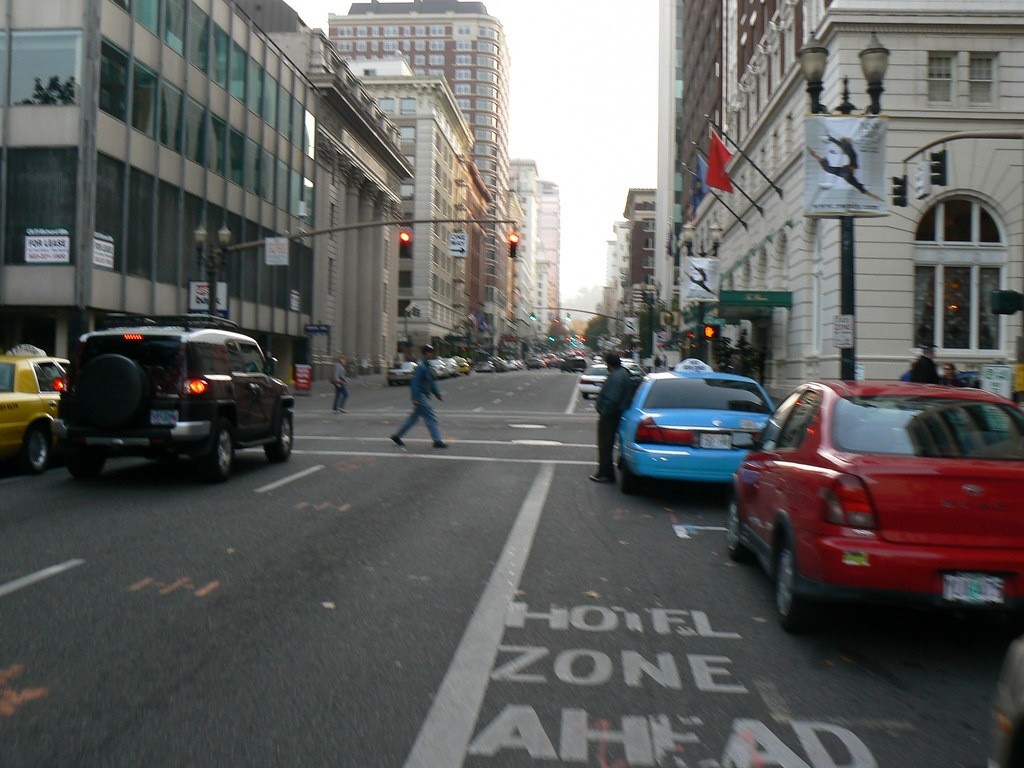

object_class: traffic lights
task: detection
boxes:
[550,336,554,341]
[399,232,409,247]
[510,235,519,258]
[529,315,536,321]
[703,324,720,339]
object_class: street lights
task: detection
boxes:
[641,279,662,358]
[683,218,723,364]
[194,222,231,316]
[796,27,892,383]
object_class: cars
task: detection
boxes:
[725,380,1024,634]
[387,351,642,385]
[610,358,778,494]
[0,345,72,473]
[578,364,612,399]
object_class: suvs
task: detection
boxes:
[56,313,295,482]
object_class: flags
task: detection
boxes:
[684,119,736,223]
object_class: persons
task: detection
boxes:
[589,352,632,483]
[721,353,739,376]
[900,342,971,431]
[654,354,662,372]
[330,355,351,415]
[390,344,448,448]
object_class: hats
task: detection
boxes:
[919,340,938,351]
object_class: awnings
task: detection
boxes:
[682,289,797,324]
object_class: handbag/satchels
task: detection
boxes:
[329,375,341,389]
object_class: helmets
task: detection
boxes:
[422,344,434,353]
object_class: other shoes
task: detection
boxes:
[390,435,404,446]
[332,410,340,414]
[433,440,449,449]
[337,407,347,413]
[588,472,616,483]
